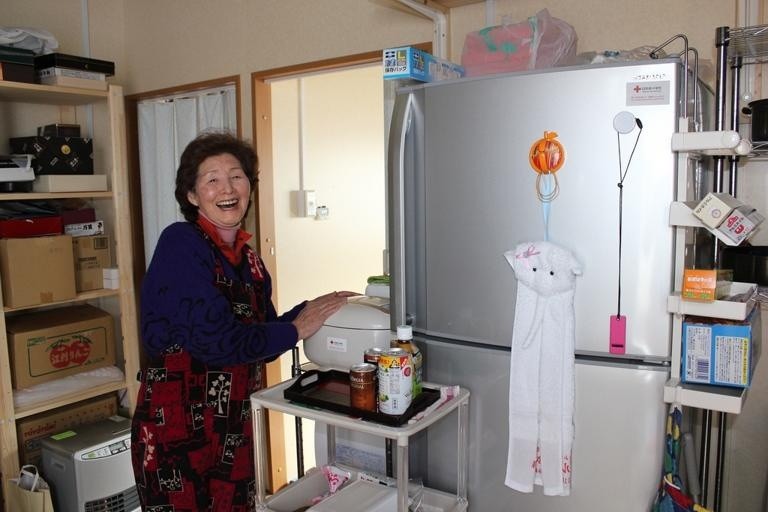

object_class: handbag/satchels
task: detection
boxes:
[4,464,55,512]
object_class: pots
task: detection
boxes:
[748,98,768,150]
[726,246,768,285]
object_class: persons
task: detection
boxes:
[129,126,366,511]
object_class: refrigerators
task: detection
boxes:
[389,59,717,287]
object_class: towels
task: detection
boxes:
[503,249,575,497]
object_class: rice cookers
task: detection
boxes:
[302,295,396,373]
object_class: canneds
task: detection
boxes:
[378,347,415,415]
[349,364,378,412]
[363,347,380,412]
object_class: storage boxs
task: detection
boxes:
[5,302,117,391]
[16,393,120,475]
[680,301,761,388]
[0,234,77,309]
[10,135,94,175]
[70,235,111,293]
[1,45,36,84]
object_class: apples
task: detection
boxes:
[50,345,69,368]
[69,341,89,365]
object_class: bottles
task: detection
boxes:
[394,326,424,404]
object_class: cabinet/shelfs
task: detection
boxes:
[0,65,142,512]
[704,28,767,512]
[249,364,472,512]
